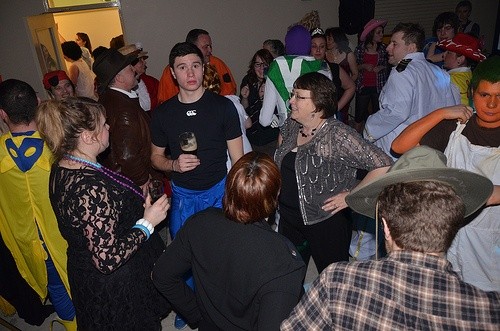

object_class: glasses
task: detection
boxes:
[290,92,310,100]
[255,63,268,68]
[131,55,148,66]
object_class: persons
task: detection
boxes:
[0,78,77,331]
[36,94,170,331]
[156,29,293,159]
[152,41,248,329]
[42,70,75,100]
[153,150,310,331]
[280,182,500,331]
[93,35,165,194]
[40,44,58,73]
[60,40,98,101]
[311,0,500,292]
[258,24,334,147]
[74,32,94,70]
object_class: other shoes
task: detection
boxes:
[174,315,187,329]
[349,231,376,261]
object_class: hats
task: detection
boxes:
[437,33,485,62]
[92,46,137,96]
[361,19,386,40]
[345,145,493,220]
[42,70,69,89]
[117,43,148,58]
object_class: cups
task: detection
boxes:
[148,180,163,201]
[179,132,197,156]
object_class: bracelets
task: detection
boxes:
[135,218,154,235]
[171,158,179,171]
[131,224,150,241]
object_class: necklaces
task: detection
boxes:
[62,154,147,203]
[298,119,324,137]
